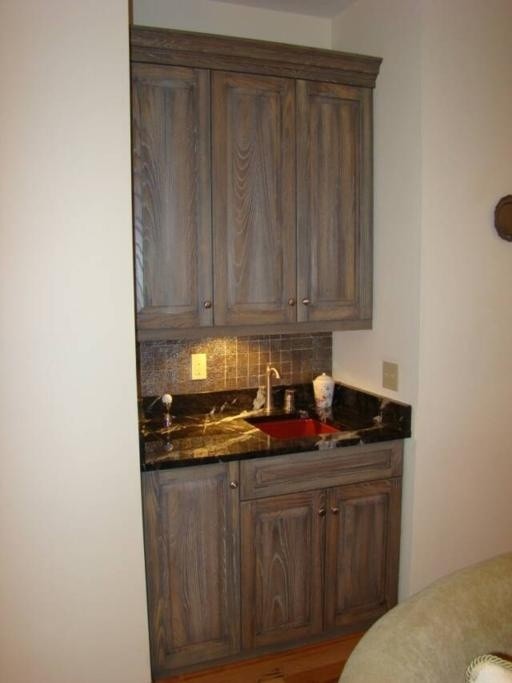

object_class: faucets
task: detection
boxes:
[265,367,281,412]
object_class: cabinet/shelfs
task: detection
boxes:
[245,440,401,663]
[141,461,242,679]
[130,58,210,339]
[213,68,373,335]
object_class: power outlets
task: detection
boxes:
[382,363,398,391]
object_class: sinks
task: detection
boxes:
[243,409,343,440]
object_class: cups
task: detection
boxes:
[312,372,336,409]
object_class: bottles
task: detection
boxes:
[161,393,175,429]
